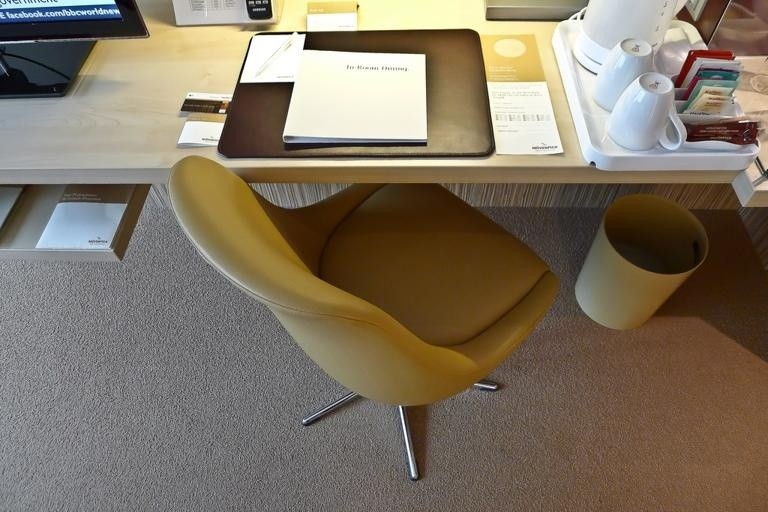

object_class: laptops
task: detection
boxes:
[215,28,496,157]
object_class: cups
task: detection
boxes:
[591,36,654,114]
[606,72,689,151]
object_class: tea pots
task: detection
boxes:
[571,0,688,76]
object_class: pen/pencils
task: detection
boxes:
[247,32,298,77]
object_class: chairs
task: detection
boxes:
[168,155,561,481]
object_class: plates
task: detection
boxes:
[552,18,762,172]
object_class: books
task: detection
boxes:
[38,182,133,250]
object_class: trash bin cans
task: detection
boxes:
[573,191,709,330]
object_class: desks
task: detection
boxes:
[731,54,768,209]
[0,19,744,185]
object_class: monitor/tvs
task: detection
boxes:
[0,1,150,99]
